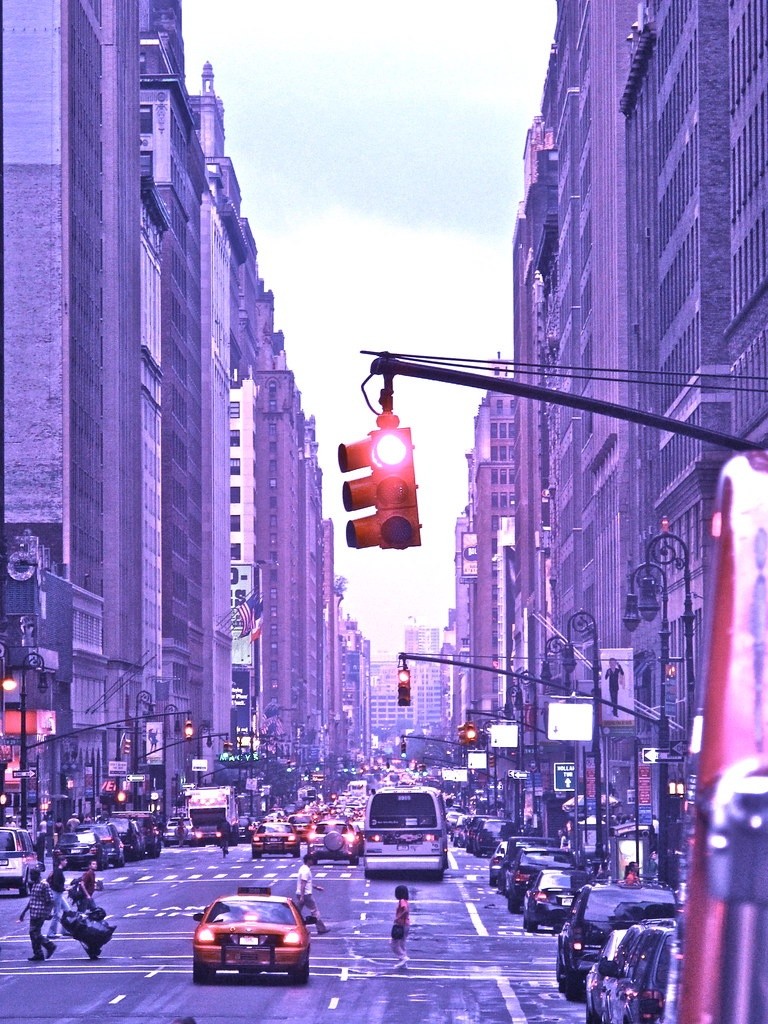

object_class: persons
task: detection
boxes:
[66,813,80,833]
[540,701,548,739]
[624,862,641,885]
[605,657,624,717]
[560,827,571,850]
[53,817,64,847]
[646,851,658,880]
[46,856,71,939]
[295,854,331,935]
[16,868,57,961]
[83,813,92,824]
[159,812,167,839]
[36,817,47,832]
[77,858,98,912]
[217,816,231,854]
[4,816,16,827]
[597,862,612,885]
[177,816,186,848]
[95,810,102,823]
[391,885,410,969]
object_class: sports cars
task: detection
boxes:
[192,886,318,986]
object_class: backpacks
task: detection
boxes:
[67,876,84,905]
[46,870,54,883]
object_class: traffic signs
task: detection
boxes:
[642,748,684,764]
[512,771,530,780]
[554,762,578,791]
[180,783,197,790]
[12,770,37,778]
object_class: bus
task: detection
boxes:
[362,786,453,881]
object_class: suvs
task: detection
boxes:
[163,817,194,848]
[111,811,161,859]
[71,822,126,868]
[0,827,40,898]
[502,846,578,915]
[497,835,558,893]
[307,820,361,865]
[550,882,683,1002]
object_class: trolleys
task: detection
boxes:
[46,913,102,960]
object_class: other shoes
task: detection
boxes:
[61,931,70,936]
[28,955,45,961]
[47,934,62,938]
[318,929,331,934]
[401,963,407,969]
[45,943,56,959]
[394,957,410,969]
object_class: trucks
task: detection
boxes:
[186,785,240,847]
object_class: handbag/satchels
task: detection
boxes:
[391,924,404,940]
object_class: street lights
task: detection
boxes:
[162,703,181,823]
[196,722,214,785]
[482,669,540,836]
[617,531,697,888]
[561,608,606,877]
[2,652,49,829]
[129,690,157,810]
[538,635,579,856]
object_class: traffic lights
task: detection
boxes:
[405,763,409,772]
[398,670,410,706]
[124,739,132,754]
[422,765,428,777]
[491,756,494,767]
[369,427,421,548]
[466,725,476,740]
[386,762,390,774]
[400,742,407,758]
[339,436,380,548]
[223,744,229,759]
[185,722,193,742]
[115,790,128,803]
[457,726,466,745]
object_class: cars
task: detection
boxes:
[522,869,599,933]
[488,840,509,887]
[251,822,301,859]
[585,917,683,1024]
[94,817,144,861]
[238,777,370,843]
[442,790,519,859]
[52,832,110,870]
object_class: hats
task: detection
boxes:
[72,813,78,816]
